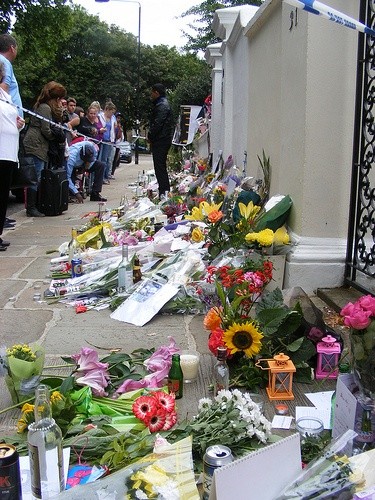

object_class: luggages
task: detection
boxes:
[36,160,68,215]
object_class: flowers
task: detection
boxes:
[0,149,375,500]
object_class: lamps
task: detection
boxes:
[316,334,340,380]
[254,352,296,400]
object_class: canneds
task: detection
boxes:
[201,444,235,500]
[71,258,82,278]
[0,444,23,500]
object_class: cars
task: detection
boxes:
[131,143,147,152]
[116,142,133,165]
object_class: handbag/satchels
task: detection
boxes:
[6,132,38,190]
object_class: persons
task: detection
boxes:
[147,83,174,201]
[23,81,67,217]
[0,34,124,251]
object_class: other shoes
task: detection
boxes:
[107,175,116,180]
[0,240,10,251]
[68,186,93,202]
[4,218,16,227]
[26,209,45,217]
[103,179,110,185]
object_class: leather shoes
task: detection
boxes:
[90,194,107,202]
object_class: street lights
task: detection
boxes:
[95,0,141,164]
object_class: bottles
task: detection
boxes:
[213,347,229,395]
[132,258,142,284]
[27,384,66,499]
[117,244,134,294]
[68,227,83,280]
[120,194,129,215]
[167,353,184,400]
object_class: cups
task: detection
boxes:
[296,417,325,445]
[178,349,200,384]
